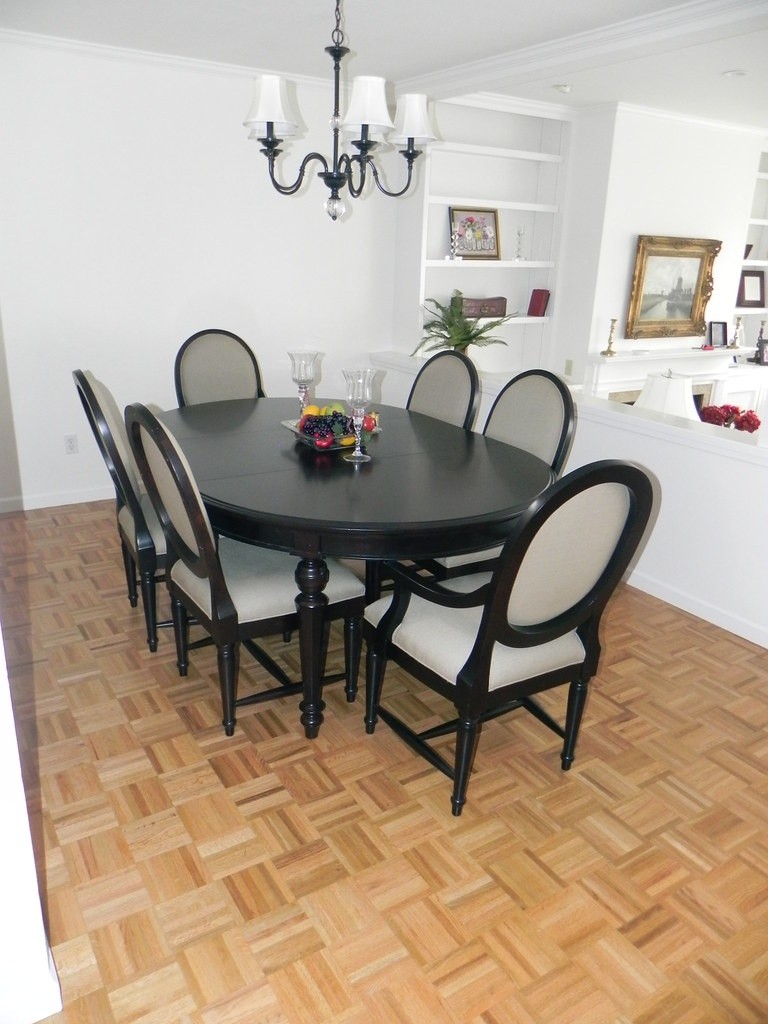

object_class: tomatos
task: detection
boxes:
[361,415,375,432]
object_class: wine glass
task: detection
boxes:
[341,368,378,462]
[287,350,318,418]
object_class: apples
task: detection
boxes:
[325,403,344,416]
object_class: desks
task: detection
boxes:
[154,398,558,739]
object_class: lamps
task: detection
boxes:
[633,368,700,422]
[244,0,437,221]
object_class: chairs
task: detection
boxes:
[72,369,225,652]
[124,402,365,736]
[175,329,266,408]
[366,369,575,605]
[364,458,653,815]
[406,350,480,430]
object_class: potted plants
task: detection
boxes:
[409,288,519,355]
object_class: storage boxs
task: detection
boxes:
[451,297,507,317]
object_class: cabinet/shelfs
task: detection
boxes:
[735,172,768,314]
[396,101,569,357]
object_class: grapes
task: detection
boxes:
[303,410,351,437]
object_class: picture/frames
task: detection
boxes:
[449,207,501,261]
[624,235,722,340]
[710,322,727,346]
[738,271,765,307]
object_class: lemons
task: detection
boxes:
[303,405,327,416]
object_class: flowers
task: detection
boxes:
[699,404,761,433]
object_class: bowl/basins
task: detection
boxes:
[280,415,383,452]
